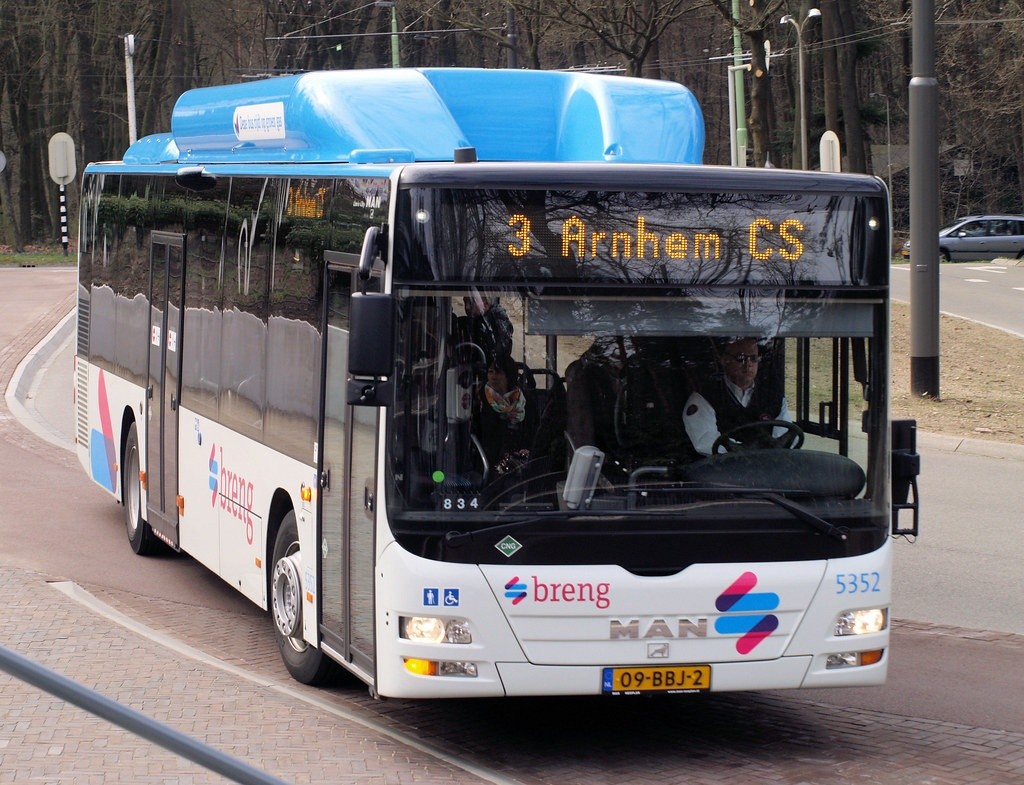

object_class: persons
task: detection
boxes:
[962,223,983,236]
[472,356,530,482]
[463,296,514,356]
[683,336,792,464]
[563,337,622,460]
[1006,229,1013,235]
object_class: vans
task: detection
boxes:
[902,216,1024,263]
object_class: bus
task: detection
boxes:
[72,67,921,699]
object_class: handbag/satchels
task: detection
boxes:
[494,449,527,479]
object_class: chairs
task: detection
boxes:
[515,362,568,465]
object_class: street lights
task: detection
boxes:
[780,8,821,171]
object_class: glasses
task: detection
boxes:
[724,351,761,363]
